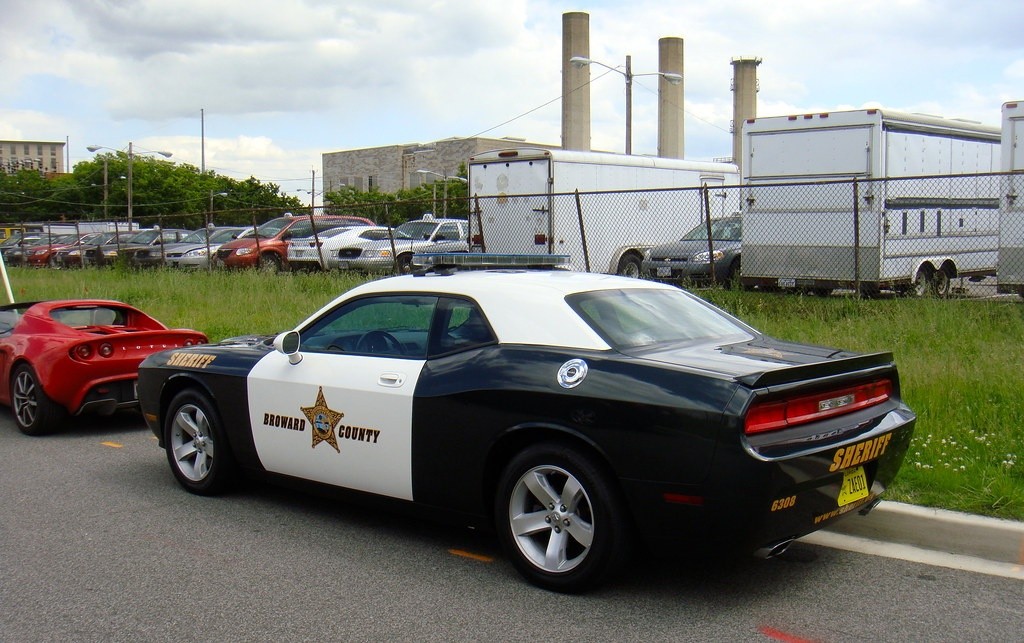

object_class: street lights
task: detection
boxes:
[209,190,228,223]
[570,56,683,156]
[86,141,172,229]
[416,169,468,219]
[311,169,346,216]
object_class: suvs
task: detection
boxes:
[215,213,381,276]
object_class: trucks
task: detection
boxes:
[995,100,1024,300]
[462,145,738,281]
[739,107,1003,300]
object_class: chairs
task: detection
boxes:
[96,308,115,326]
[730,228,741,239]
[467,309,493,345]
[712,229,725,239]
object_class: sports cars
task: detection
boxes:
[0,299,208,437]
[131,269,919,596]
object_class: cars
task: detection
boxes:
[0,224,280,274]
[285,223,412,278]
[641,211,742,291]
[337,217,468,274]
